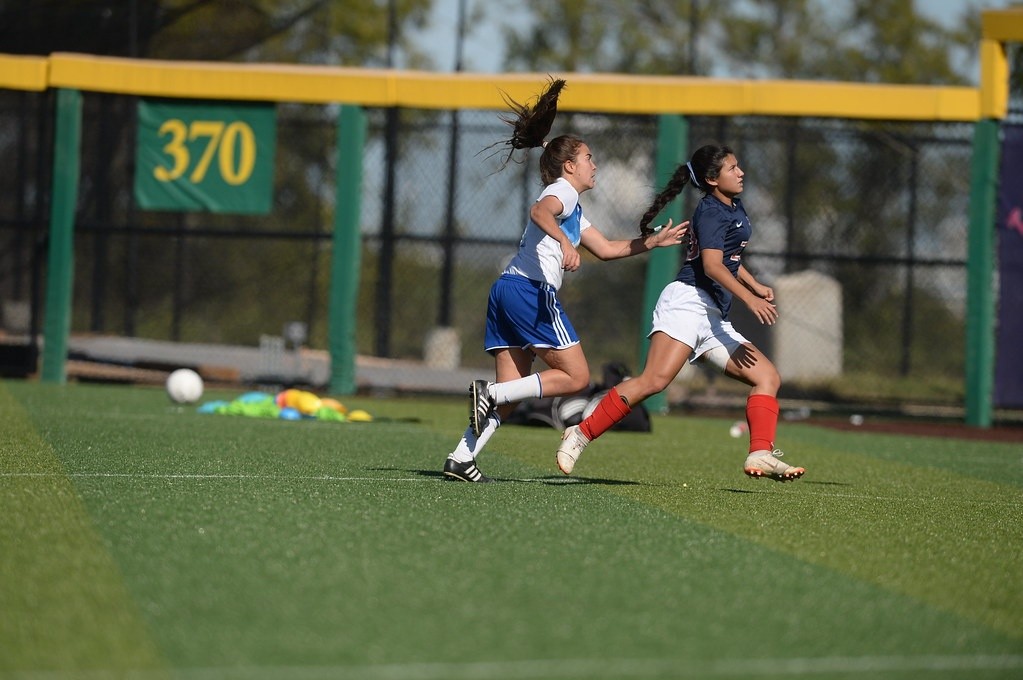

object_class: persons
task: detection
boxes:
[555,144,806,482]
[442,79,689,482]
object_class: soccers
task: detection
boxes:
[166,369,205,404]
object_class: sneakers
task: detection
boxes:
[469,380,496,437]
[744,449,804,483]
[444,453,489,483]
[555,425,590,475]
